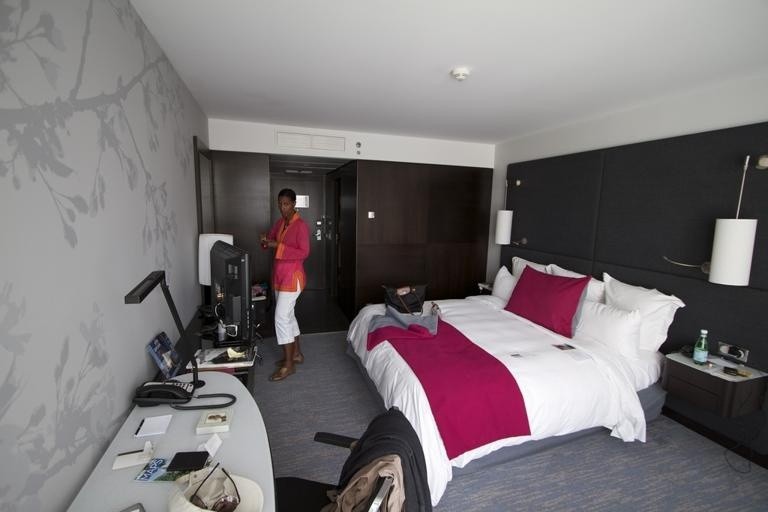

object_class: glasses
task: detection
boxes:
[188,463,241,512]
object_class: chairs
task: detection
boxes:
[266,404,406,511]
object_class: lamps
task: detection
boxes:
[196,231,235,315]
[701,151,768,287]
[123,270,206,390]
[492,176,530,248]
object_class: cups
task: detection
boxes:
[217,323,227,341]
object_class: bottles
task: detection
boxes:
[692,328,710,365]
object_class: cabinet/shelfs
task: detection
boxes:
[180,329,263,398]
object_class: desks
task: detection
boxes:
[62,369,278,512]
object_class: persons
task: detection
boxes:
[258,187,310,382]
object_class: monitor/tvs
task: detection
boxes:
[210,240,251,346]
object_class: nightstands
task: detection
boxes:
[659,346,767,422]
[477,283,492,295]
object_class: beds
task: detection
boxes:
[344,252,688,509]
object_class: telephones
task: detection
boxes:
[132,381,195,407]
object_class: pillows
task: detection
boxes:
[490,255,687,368]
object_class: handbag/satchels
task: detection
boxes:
[381,281,431,316]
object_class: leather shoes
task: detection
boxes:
[275,353,305,367]
[271,363,297,381]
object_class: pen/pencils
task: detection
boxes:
[118,450,144,456]
[135,419,144,435]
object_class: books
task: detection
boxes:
[110,446,154,470]
[134,412,174,436]
[195,406,235,435]
[134,455,223,484]
[167,451,209,470]
[185,345,258,369]
